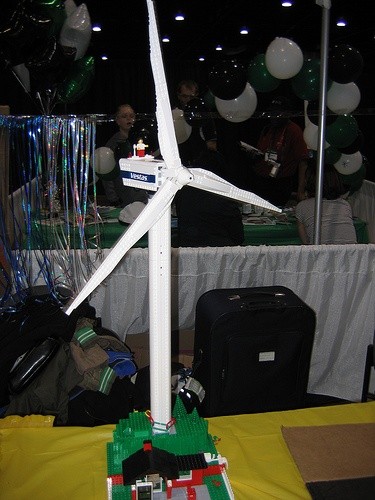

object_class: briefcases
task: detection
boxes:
[194,284,317,416]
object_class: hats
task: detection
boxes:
[272,96,288,106]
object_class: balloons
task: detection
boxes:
[0,0,97,105]
[153,0,375,195]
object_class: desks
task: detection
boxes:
[21,205,371,249]
[0,401,375,500]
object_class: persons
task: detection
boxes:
[106,103,144,151]
[251,117,307,208]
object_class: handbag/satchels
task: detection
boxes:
[1,285,191,427]
